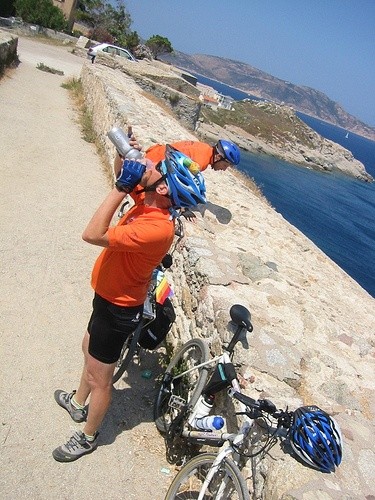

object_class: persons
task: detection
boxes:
[52,123,207,463]
[160,139,240,173]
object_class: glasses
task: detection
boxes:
[156,159,171,197]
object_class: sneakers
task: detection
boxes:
[53,430,100,462]
[54,390,87,422]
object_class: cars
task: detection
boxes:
[87,43,138,64]
[5,16,22,26]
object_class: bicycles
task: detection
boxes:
[153,304,293,500]
[113,253,175,386]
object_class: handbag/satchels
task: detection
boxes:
[139,298,177,351]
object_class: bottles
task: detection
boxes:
[189,395,215,428]
[194,415,225,431]
[108,126,141,160]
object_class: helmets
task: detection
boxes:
[215,139,241,165]
[165,144,206,208]
[289,404,343,473]
